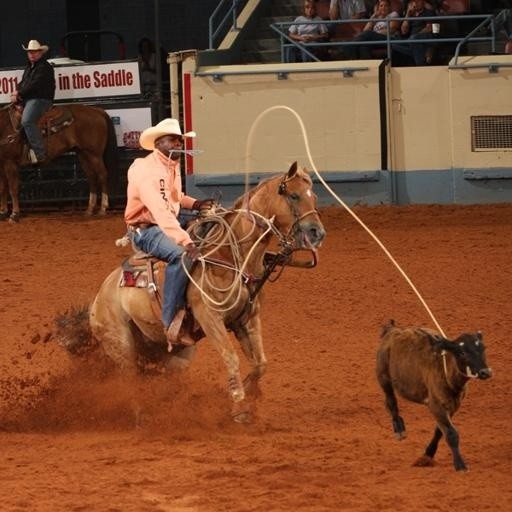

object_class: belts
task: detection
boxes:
[130,224,151,229]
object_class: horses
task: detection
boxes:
[89,160,326,431]
[375,317,492,473]
[0,104,117,224]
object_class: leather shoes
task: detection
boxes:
[179,330,195,346]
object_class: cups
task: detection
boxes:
[432,23,441,33]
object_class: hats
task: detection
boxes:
[21,39,49,53]
[139,118,196,151]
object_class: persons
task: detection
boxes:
[137,39,158,87]
[10,39,56,168]
[284,0,512,66]
[123,118,214,347]
